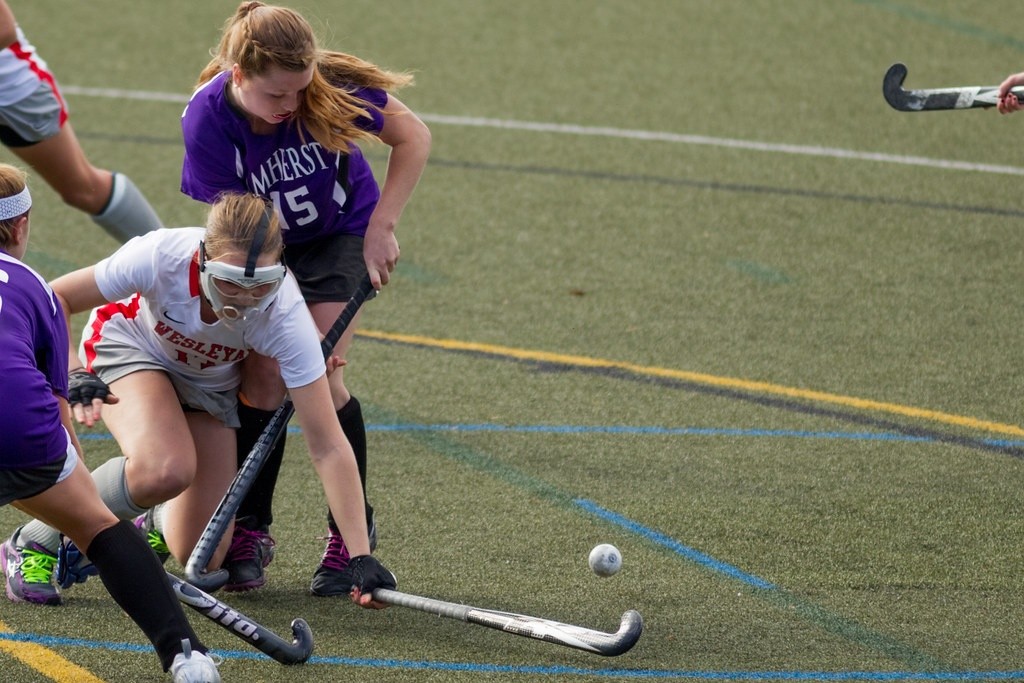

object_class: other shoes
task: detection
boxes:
[170,638,224,683]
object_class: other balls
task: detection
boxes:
[589,543,623,576]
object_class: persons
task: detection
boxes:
[179,1,432,599]
[0,193,398,612]
[0,1,168,251]
[996,71,1024,115]
[0,161,222,683]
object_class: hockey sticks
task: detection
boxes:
[166,273,373,666]
[370,586,643,656]
[882,63,1024,112]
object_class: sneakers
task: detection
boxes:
[220,524,276,590]
[1,524,61,608]
[133,506,171,565]
[310,506,376,595]
